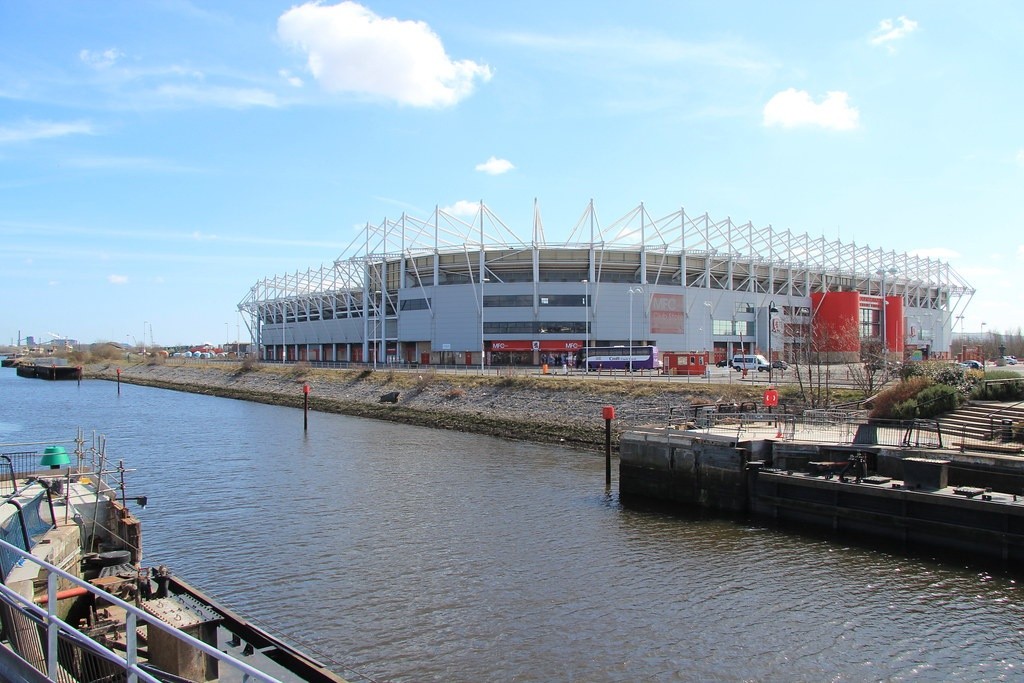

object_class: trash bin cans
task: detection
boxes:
[1001,419,1013,442]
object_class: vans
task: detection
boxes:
[733,354,773,372]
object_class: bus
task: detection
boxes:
[580,346,659,373]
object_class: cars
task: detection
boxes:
[772,360,788,371]
[716,359,734,368]
[962,360,983,371]
[988,355,1018,366]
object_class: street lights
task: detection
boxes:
[373,291,383,372]
[627,287,634,373]
[582,280,589,374]
[481,278,489,375]
[768,300,778,425]
[225,322,229,360]
[143,320,148,362]
[701,300,710,355]
[236,324,240,359]
